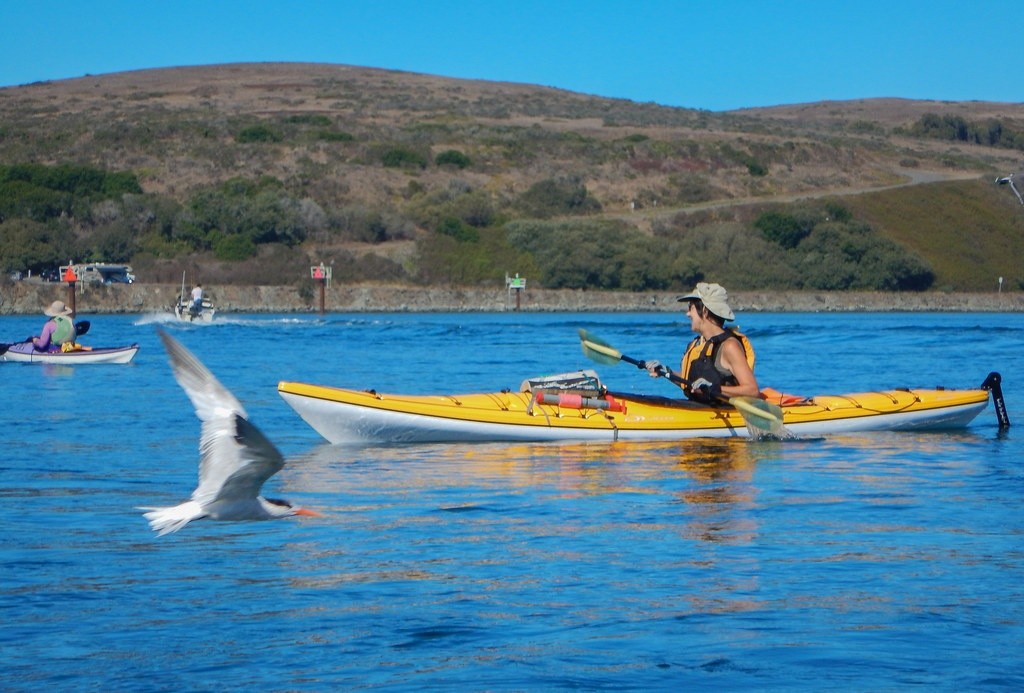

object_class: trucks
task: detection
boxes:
[82,265,135,285]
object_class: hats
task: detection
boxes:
[677,282,735,322]
[43,300,73,318]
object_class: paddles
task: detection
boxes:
[575,325,784,429]
[0,320,91,358]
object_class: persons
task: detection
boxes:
[32,300,77,353]
[39,265,58,282]
[191,283,202,318]
[644,281,759,411]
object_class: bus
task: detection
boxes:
[58,263,105,282]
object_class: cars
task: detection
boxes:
[41,270,58,282]
[10,271,24,280]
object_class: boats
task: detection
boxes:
[175,267,216,325]
[0,341,141,368]
[274,377,990,449]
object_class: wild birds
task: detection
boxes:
[132,329,328,538]
